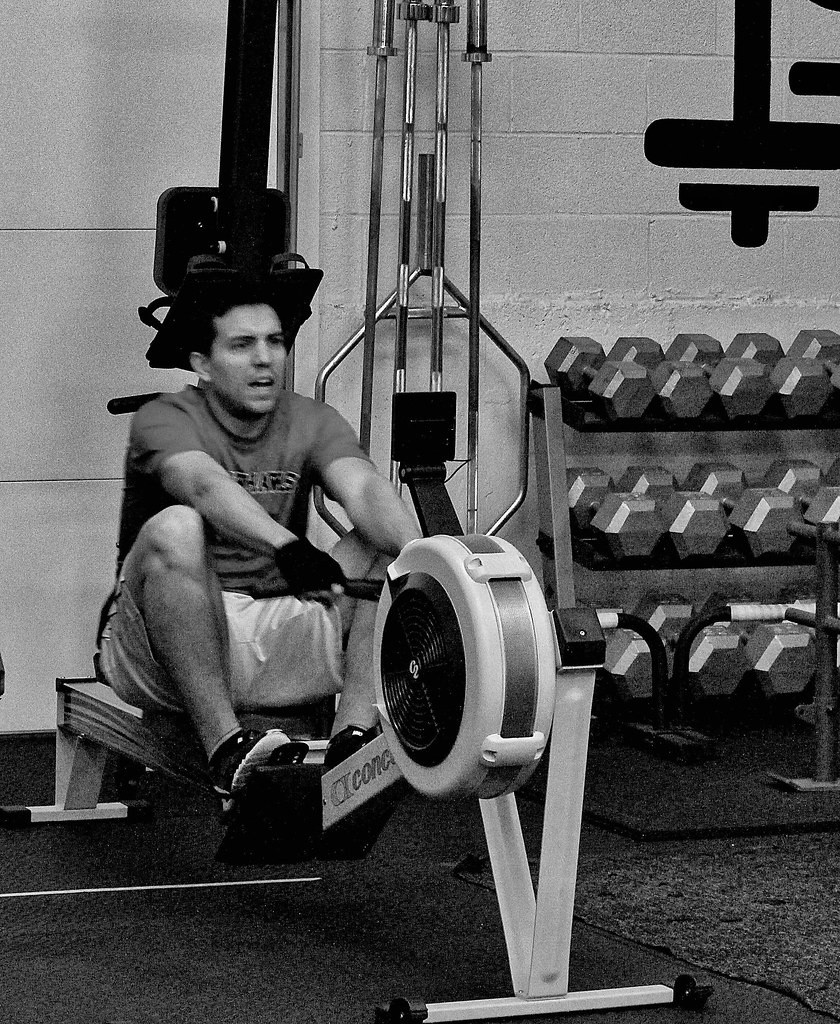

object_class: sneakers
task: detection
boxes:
[210,726,290,811]
[323,726,379,771]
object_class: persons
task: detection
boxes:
[105,294,423,813]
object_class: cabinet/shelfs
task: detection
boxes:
[524,326,840,725]
